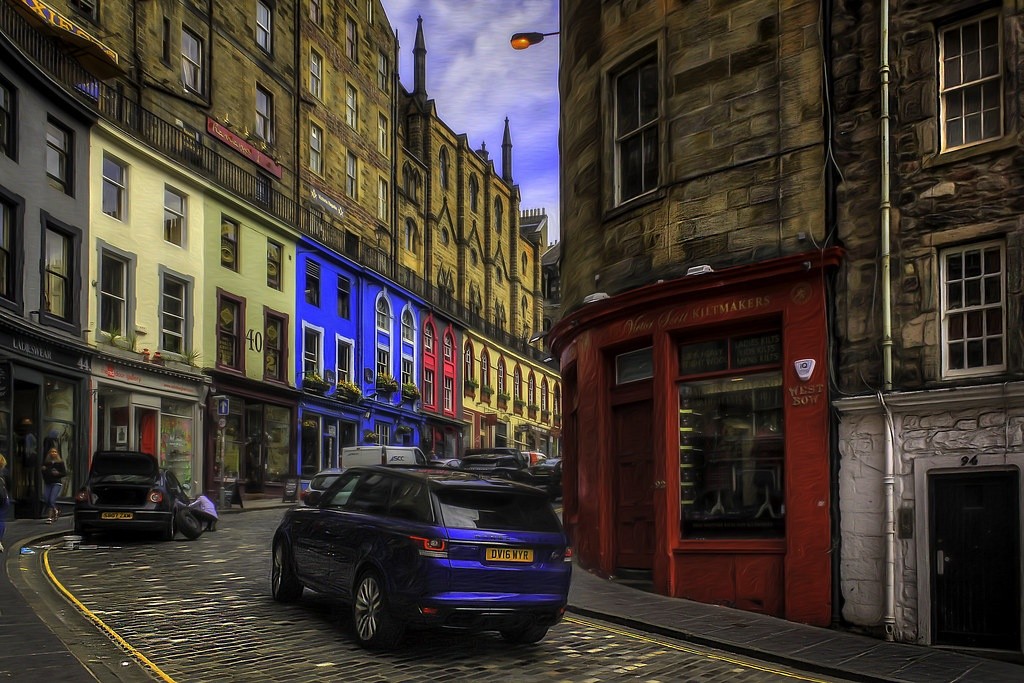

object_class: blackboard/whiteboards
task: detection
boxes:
[216,482,243,506]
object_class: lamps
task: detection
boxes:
[510,31,559,50]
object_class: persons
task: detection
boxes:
[429,451,438,460]
[187,494,218,531]
[0,454,8,552]
[42,449,62,523]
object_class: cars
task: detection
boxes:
[298,466,344,508]
[428,447,563,500]
[73,449,190,540]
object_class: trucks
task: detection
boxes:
[341,444,429,472]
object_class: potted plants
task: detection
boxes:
[303,373,331,391]
[337,380,361,401]
[376,372,398,391]
[303,420,316,430]
[465,378,562,420]
[402,382,420,399]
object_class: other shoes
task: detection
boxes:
[53,510,58,520]
[46,518,53,523]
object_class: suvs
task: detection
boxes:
[270,460,573,652]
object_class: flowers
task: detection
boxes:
[364,432,377,443]
